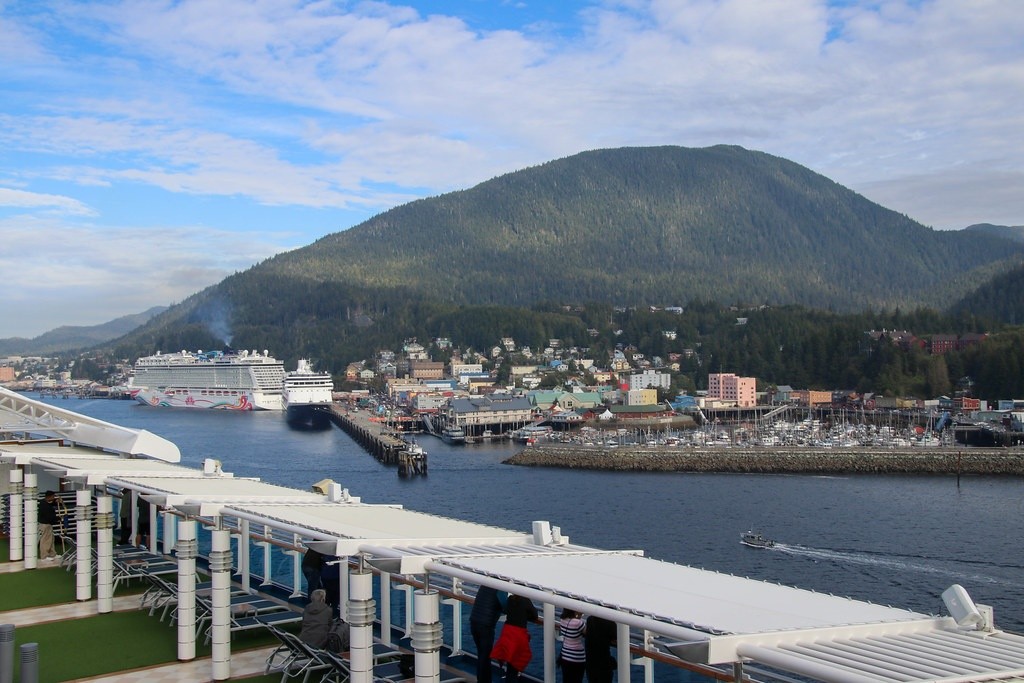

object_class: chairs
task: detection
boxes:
[57,532,466,682]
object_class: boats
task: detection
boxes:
[282,358,335,414]
[509,414,989,450]
[739,530,776,550]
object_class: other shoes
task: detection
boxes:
[332,613,340,621]
[52,554,62,559]
[116,544,120,546]
[42,557,54,562]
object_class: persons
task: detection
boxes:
[321,555,340,610]
[37,490,62,561]
[583,603,618,683]
[298,589,332,650]
[137,492,163,551]
[489,594,544,683]
[559,598,588,683]
[301,537,325,600]
[470,584,508,683]
[120,488,134,548]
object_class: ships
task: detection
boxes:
[129,348,288,410]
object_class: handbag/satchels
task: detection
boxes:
[397,654,415,677]
[556,653,562,669]
[328,624,347,653]
